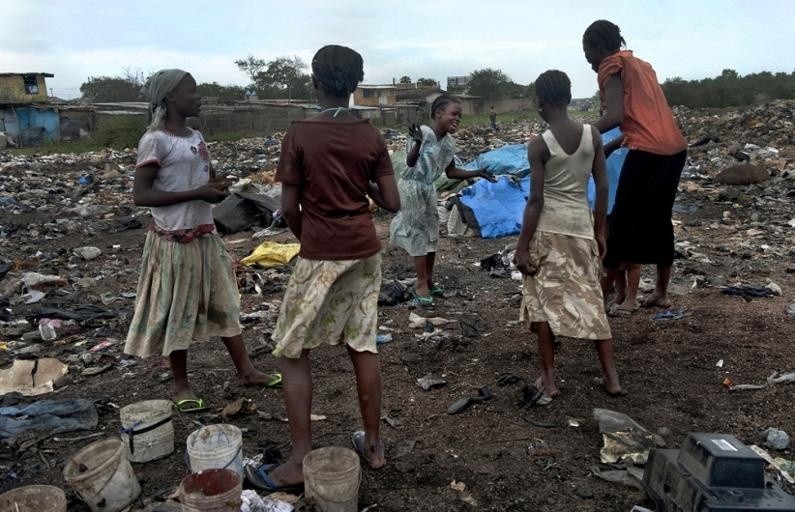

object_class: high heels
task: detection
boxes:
[447,385,494,415]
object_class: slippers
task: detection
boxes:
[536,377,561,397]
[171,393,211,413]
[245,462,303,492]
[412,286,444,310]
[245,372,284,389]
[592,377,628,396]
[604,286,674,318]
[351,430,388,470]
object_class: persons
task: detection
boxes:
[124,68,285,413]
[510,68,623,398]
[581,17,689,320]
[585,119,631,314]
[388,93,500,312]
[488,104,499,132]
[244,43,403,494]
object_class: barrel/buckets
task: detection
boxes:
[0,485,67,512]
[62,437,141,512]
[303,448,362,512]
[177,469,243,512]
[121,399,174,463]
[186,423,244,477]
